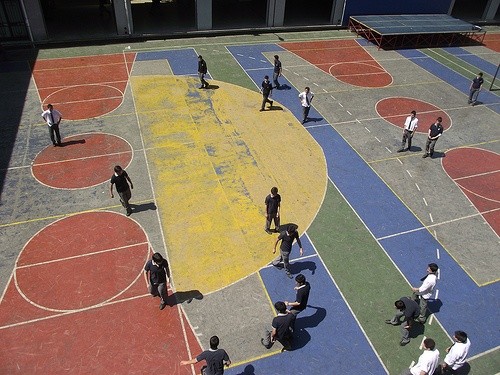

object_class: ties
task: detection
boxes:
[51,111,55,124]
[420,273,436,281]
[408,119,413,129]
[306,94,310,106]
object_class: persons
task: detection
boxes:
[298,86,314,125]
[180,336,232,375]
[466,72,484,107]
[386,297,421,347]
[110,165,133,216]
[144,253,171,310]
[41,104,63,147]
[259,76,273,111]
[261,301,301,349]
[400,339,440,375]
[408,262,438,327]
[283,273,311,318]
[272,224,303,279]
[271,55,282,90]
[263,186,282,234]
[437,329,471,375]
[197,55,209,89]
[421,116,443,158]
[397,110,419,153]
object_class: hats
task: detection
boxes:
[264,75,269,79]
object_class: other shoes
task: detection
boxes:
[399,340,410,346]
[205,83,209,88]
[385,319,392,324]
[265,228,271,234]
[428,153,433,157]
[261,338,270,349]
[127,209,132,216]
[276,264,282,270]
[275,228,280,233]
[396,148,405,152]
[199,85,204,89]
[159,303,166,310]
[285,271,293,278]
[405,148,412,151]
[423,153,428,158]
